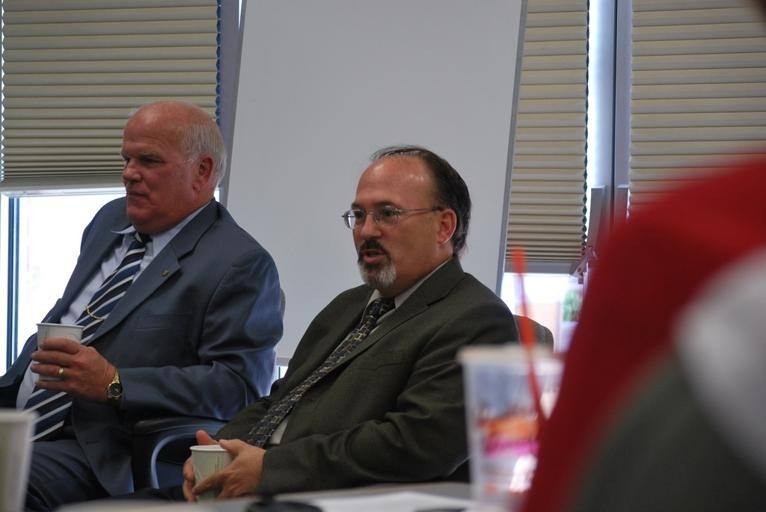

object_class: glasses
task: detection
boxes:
[341,204,437,228]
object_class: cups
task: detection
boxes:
[460,347,561,499]
[0,411,38,511]
[190,445,234,500]
[35,323,84,381]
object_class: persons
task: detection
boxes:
[116,147,518,502]
[0,100,284,512]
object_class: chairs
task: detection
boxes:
[127,312,558,494]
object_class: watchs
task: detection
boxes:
[106,369,123,401]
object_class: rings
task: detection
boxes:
[58,368,64,377]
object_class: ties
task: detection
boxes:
[23,232,153,442]
[240,297,396,447]
